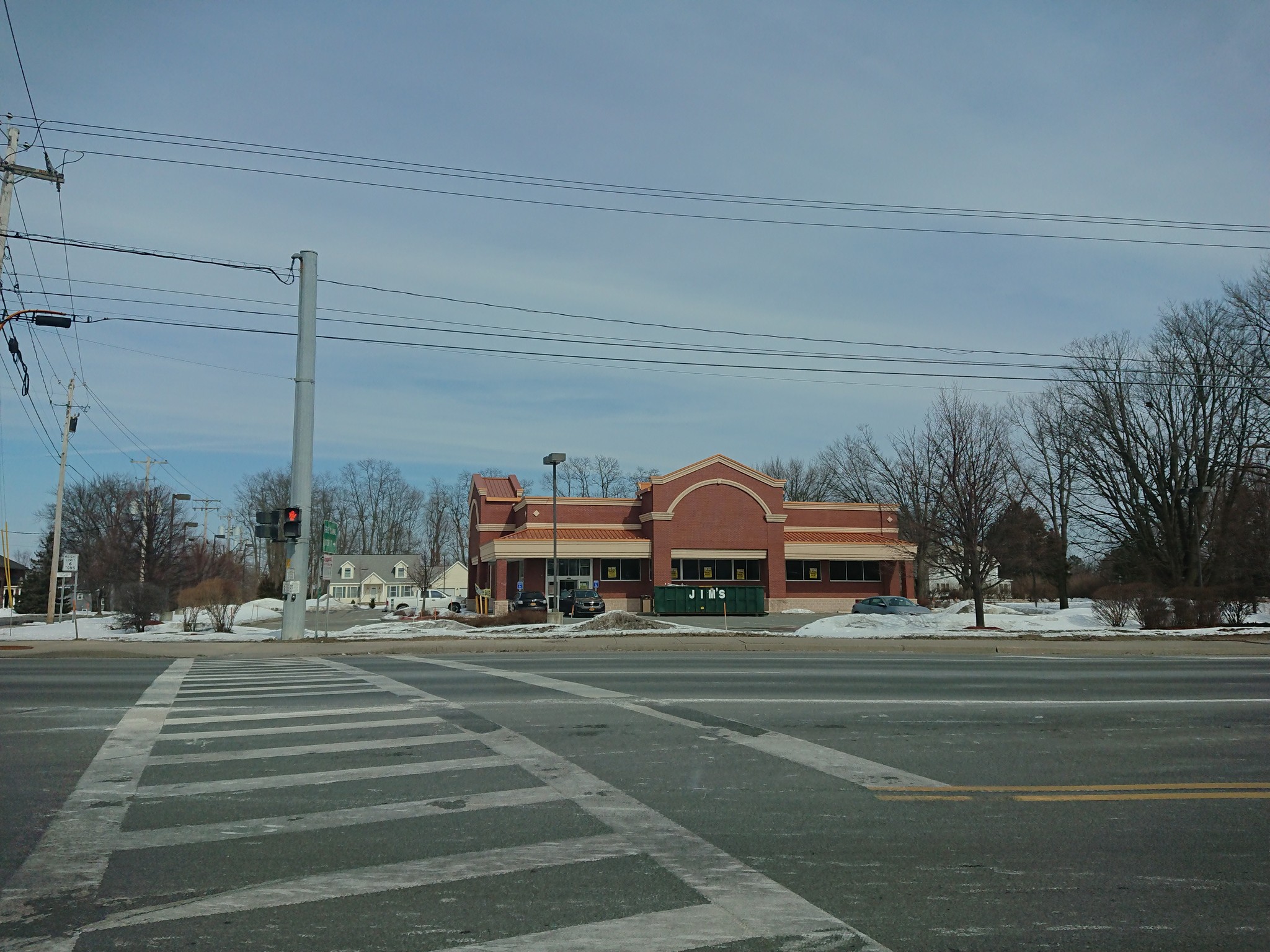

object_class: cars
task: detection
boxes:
[559,588,605,616]
[509,591,549,612]
[851,596,931,615]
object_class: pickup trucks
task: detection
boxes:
[385,589,467,613]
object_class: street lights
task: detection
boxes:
[543,453,567,625]
[158,494,191,621]
[442,553,448,593]
[175,522,198,616]
[207,535,229,580]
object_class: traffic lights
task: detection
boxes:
[282,507,301,537]
[255,510,278,538]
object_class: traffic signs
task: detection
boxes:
[323,519,339,556]
